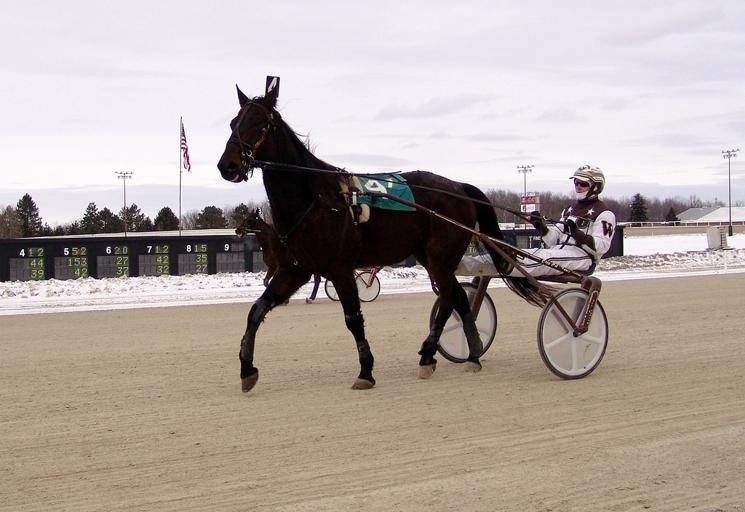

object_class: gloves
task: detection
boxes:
[530,211,542,228]
[564,218,576,234]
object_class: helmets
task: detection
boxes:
[569,165,605,193]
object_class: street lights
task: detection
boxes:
[720,146,743,238]
[515,161,536,229]
[114,169,135,237]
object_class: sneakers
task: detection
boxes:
[501,232,517,274]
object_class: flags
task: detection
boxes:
[180,118,191,172]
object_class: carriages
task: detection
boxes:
[222,71,622,398]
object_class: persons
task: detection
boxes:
[447,166,618,278]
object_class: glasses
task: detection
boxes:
[575,179,589,187]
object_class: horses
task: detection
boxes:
[215,83,562,393]
